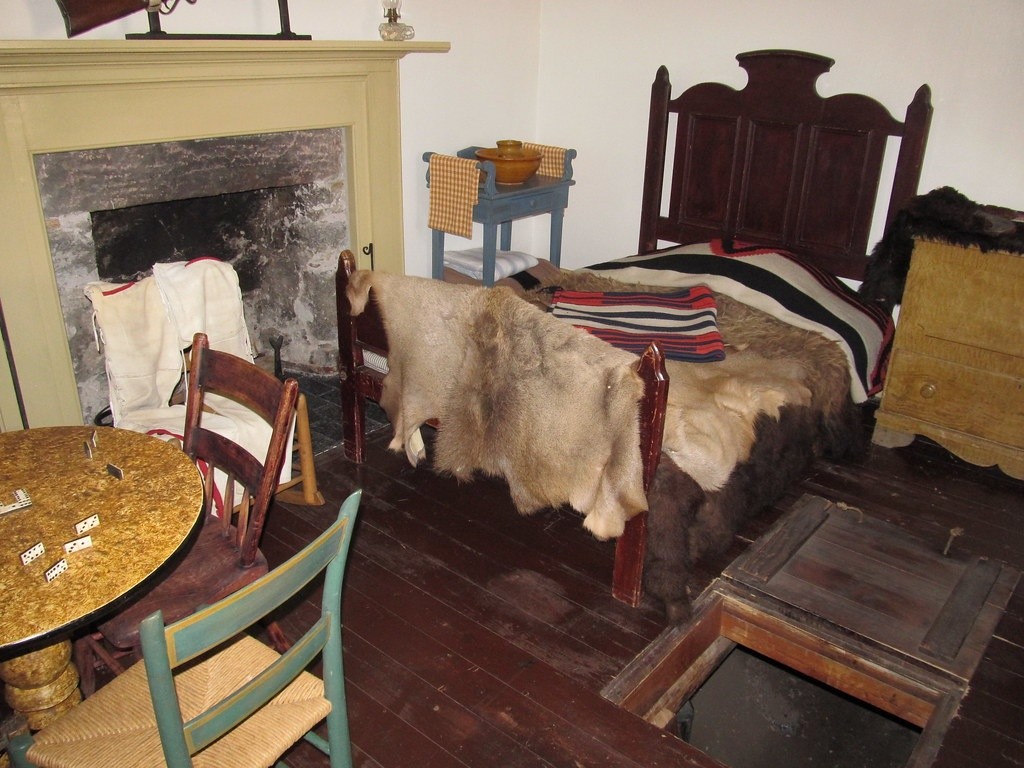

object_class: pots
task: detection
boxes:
[474,139,545,185]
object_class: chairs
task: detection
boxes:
[8,488,364,768]
[83,257,325,518]
[68,331,300,699]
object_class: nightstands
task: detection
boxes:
[872,205,1024,481]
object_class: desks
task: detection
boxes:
[0,425,210,741]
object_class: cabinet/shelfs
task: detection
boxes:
[421,140,577,291]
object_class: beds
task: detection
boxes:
[331,48,934,611]
